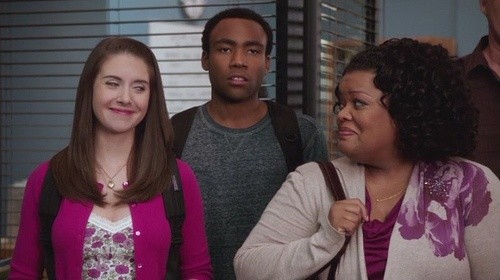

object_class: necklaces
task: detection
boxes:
[376,187,408,202]
[95,159,127,189]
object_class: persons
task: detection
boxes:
[166,7,328,280]
[233,39,500,280]
[446,0,500,180]
[8,37,214,280]
